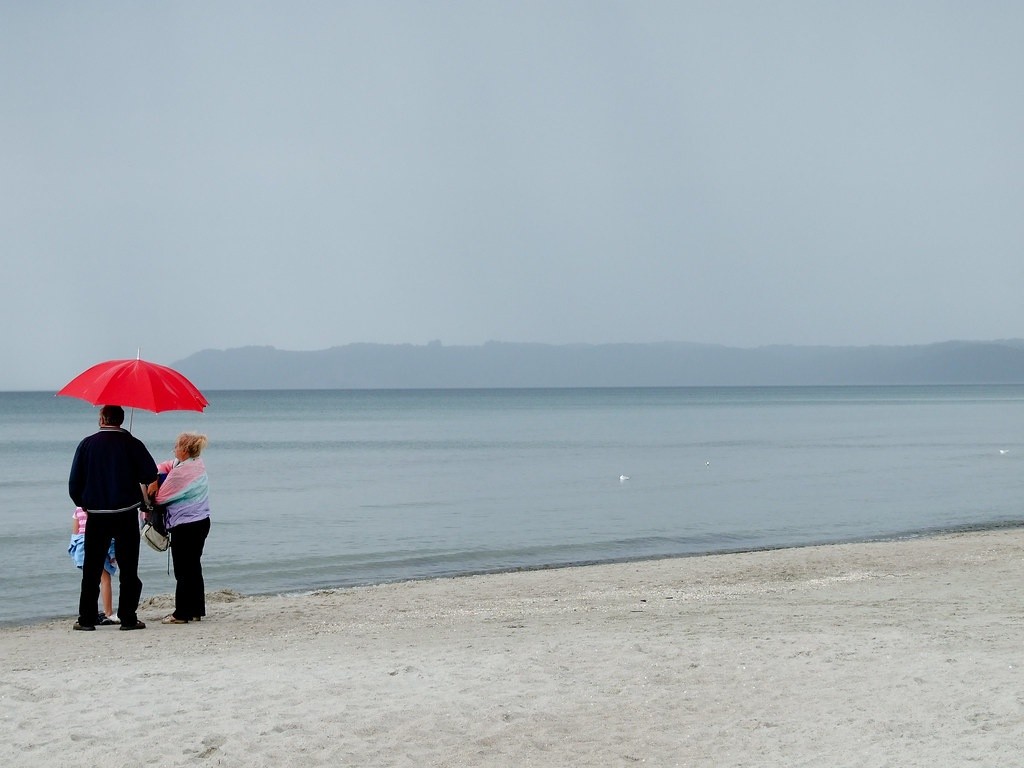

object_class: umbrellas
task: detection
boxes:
[56,349,209,432]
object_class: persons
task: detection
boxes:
[140,434,212,623]
[69,505,121,625]
[69,405,158,630]
